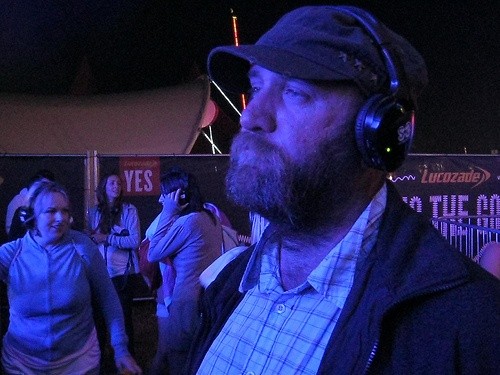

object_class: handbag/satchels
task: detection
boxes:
[137,238,162,291]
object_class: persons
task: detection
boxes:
[142,202,237,359]
[87,172,141,361]
[194,6,500,375]
[0,177,143,375]
[5,170,54,233]
[480,244,500,278]
[148,169,222,351]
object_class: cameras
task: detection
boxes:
[113,229,130,236]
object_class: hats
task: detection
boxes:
[207,7,428,113]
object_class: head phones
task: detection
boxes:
[179,168,190,205]
[323,6,415,172]
[19,179,73,230]
